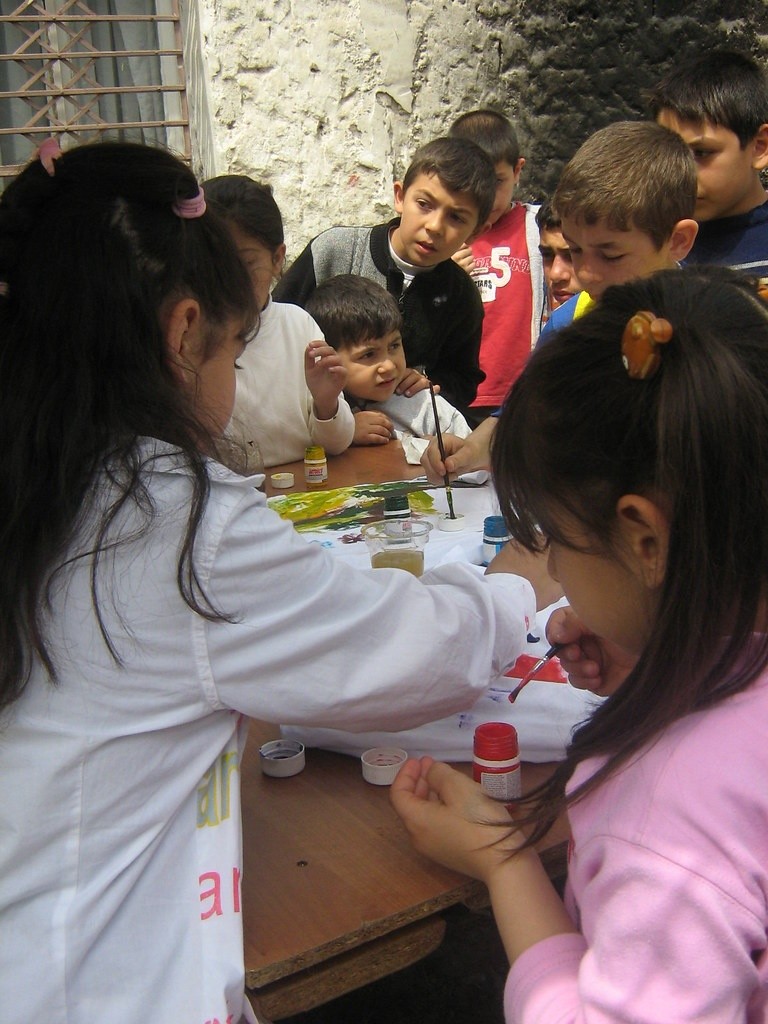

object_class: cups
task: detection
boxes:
[360,519,433,577]
[243,440,266,493]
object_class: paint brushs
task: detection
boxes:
[507,642,563,704]
[428,380,455,519]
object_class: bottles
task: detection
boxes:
[471,721,523,811]
[482,515,513,568]
[304,446,328,488]
[384,493,411,544]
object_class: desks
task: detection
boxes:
[238,427,572,1024]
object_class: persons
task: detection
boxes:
[420,120,767,490]
[200,175,354,472]
[299,273,489,484]
[0,134,564,1024]
[269,135,498,417]
[442,110,551,428]
[534,190,587,309]
[648,46,768,304]
[386,271,768,1024]
